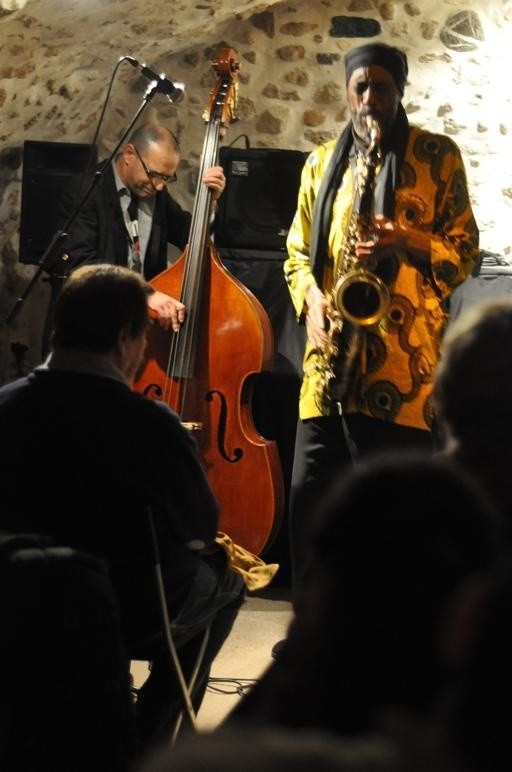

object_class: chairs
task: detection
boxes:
[0,497,216,738]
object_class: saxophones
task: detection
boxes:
[316,113,390,417]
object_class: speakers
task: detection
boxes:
[213,147,303,251]
[19,140,98,264]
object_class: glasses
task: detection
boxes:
[133,144,177,184]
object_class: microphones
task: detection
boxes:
[125,55,185,104]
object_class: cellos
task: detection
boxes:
[130,48,288,567]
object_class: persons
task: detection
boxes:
[0,262,245,747]
[265,42,483,663]
[0,526,135,772]
[48,122,227,335]
[136,452,507,771]
[425,291,512,508]
